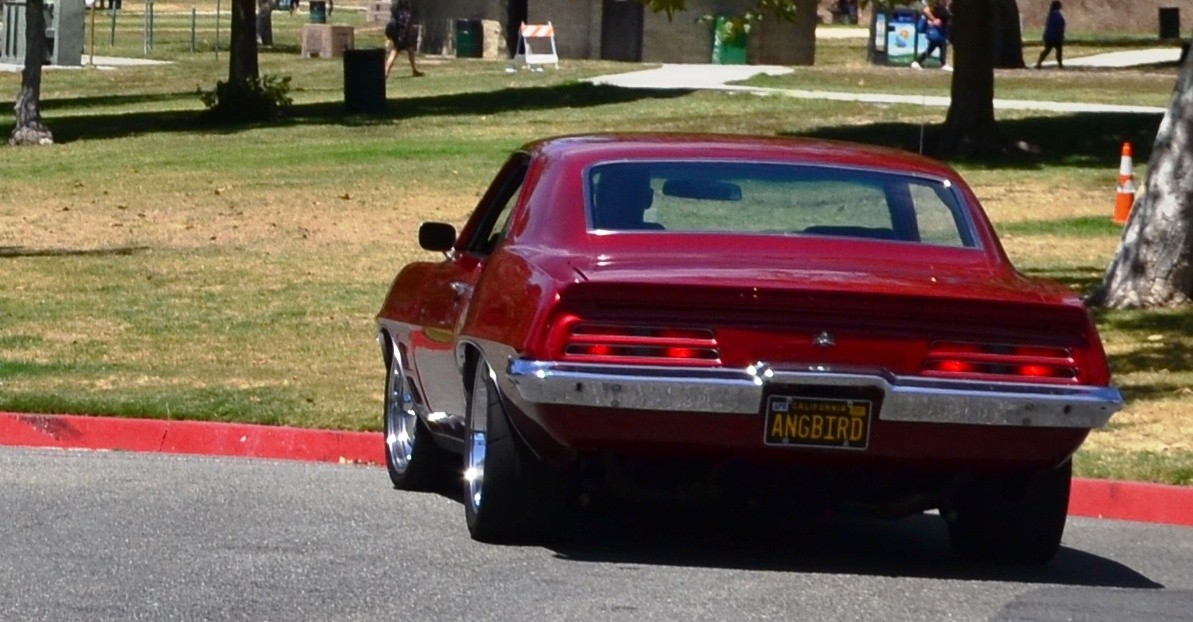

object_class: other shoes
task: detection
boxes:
[413,71,425,76]
[911,60,923,71]
[941,65,954,72]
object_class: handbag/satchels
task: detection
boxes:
[385,22,399,38]
[926,25,946,42]
[917,13,928,33]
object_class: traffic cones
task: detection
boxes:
[1110,144,1139,224]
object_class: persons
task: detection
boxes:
[385,0,426,77]
[597,168,665,230]
[1035,0,1069,70]
[911,0,954,71]
[289,0,299,16]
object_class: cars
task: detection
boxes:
[376,131,1124,562]
